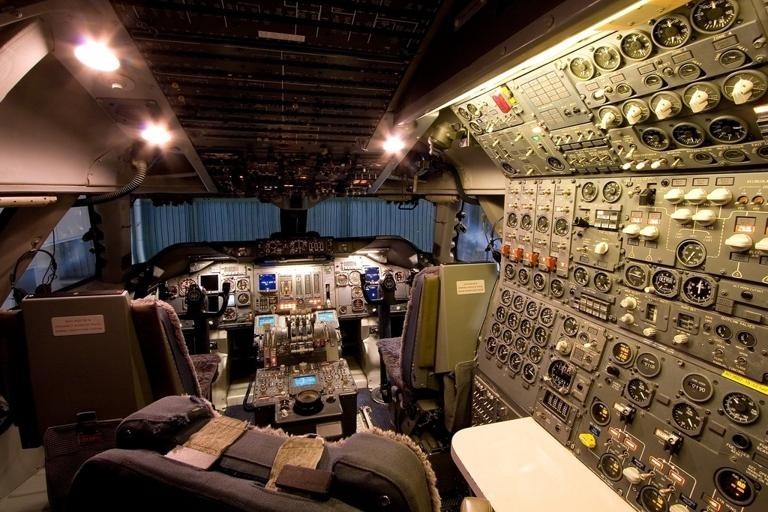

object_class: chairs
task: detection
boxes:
[1,289,220,510]
[66,395,441,510]
[377,258,499,435]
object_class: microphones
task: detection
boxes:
[481,212,494,252]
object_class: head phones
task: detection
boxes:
[10,247,57,306]
[485,216,503,262]
